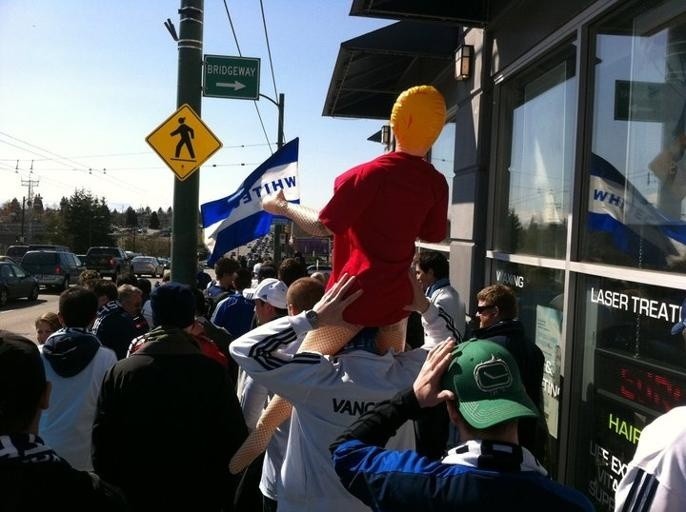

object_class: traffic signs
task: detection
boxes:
[201,53,259,100]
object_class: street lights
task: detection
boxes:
[20,194,42,239]
[87,212,105,246]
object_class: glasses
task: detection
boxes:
[477,305,496,314]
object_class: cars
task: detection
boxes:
[240,232,274,261]
[0,244,171,308]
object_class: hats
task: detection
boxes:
[670,297,686,336]
[151,281,194,328]
[242,278,288,310]
[444,336,539,430]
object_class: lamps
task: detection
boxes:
[453,43,474,83]
[380,125,390,146]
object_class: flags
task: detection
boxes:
[201,136,299,269]
[586,152,686,271]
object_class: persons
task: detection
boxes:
[227,83,449,474]
